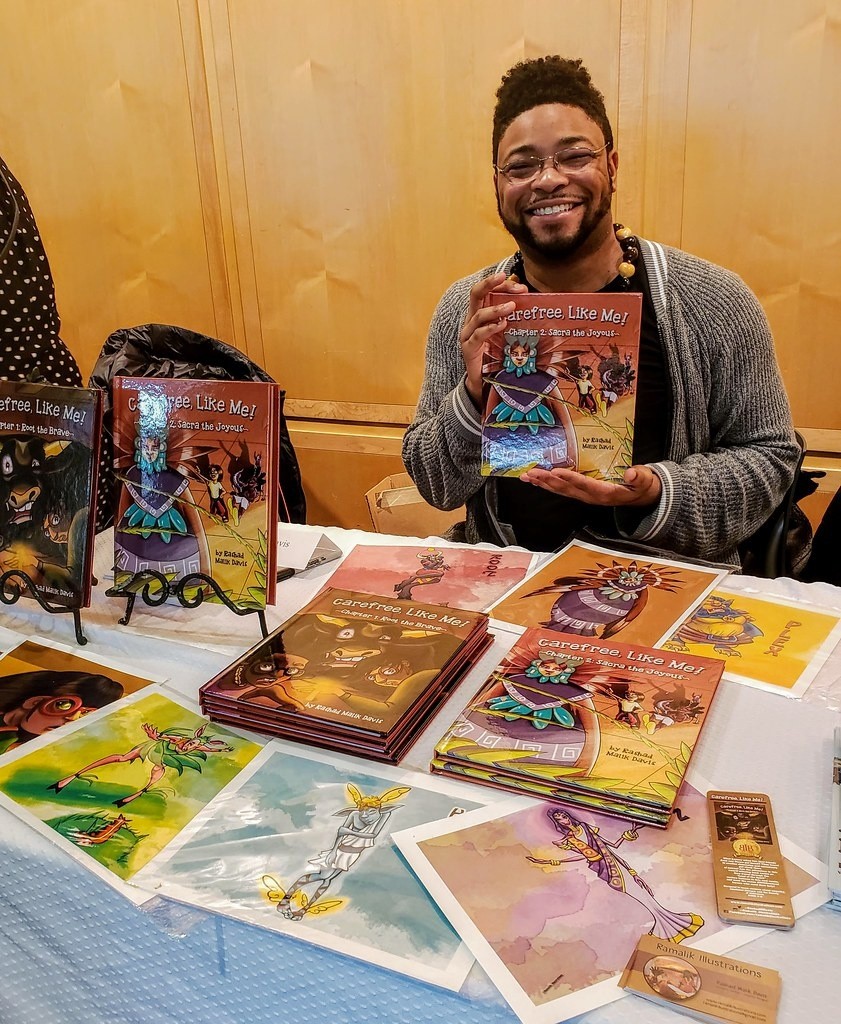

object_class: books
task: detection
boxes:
[429,627,726,830]
[198,587,494,766]
[481,291,643,483]
[111,375,279,610]
[0,379,103,609]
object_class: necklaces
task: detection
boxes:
[507,223,638,293]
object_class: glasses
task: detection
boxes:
[492,142,612,183]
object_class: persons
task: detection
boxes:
[0,157,114,534]
[400,55,800,574]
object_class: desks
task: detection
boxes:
[0,476,841,1022]
